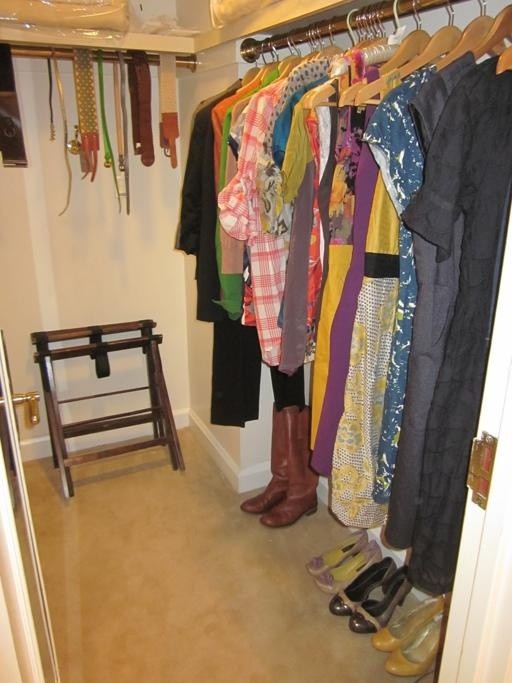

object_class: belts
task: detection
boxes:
[48,44,178,217]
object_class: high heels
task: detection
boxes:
[305,529,412,634]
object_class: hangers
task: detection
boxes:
[224,1,512,93]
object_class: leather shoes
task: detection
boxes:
[371,589,453,678]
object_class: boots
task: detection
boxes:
[240,402,297,514]
[260,405,319,528]
